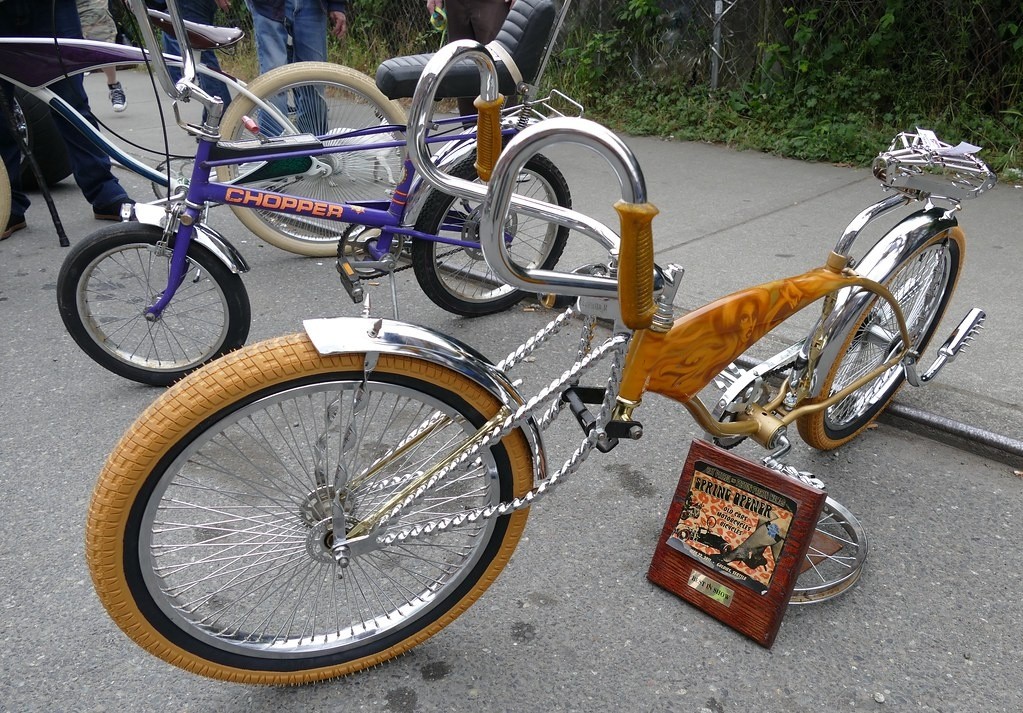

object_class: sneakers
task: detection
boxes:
[107,82,127,111]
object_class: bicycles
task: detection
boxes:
[1,0,997,689]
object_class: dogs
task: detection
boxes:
[310,108,398,188]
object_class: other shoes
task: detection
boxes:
[92,197,137,221]
[1,215,27,238]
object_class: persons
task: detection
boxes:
[108,0,132,45]
[0,0,138,240]
[244,0,346,138]
[164,0,231,128]
[426,0,530,183]
[75,0,127,113]
